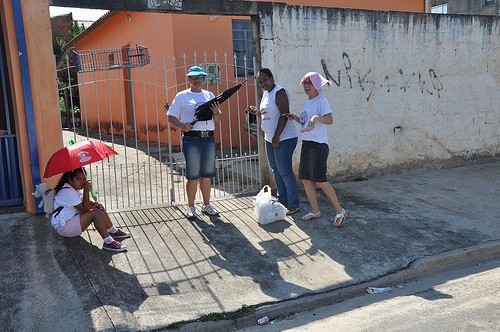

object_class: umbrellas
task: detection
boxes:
[190,79,250,129]
[43,139,118,202]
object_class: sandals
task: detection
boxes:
[332,208,348,226]
[302,211,321,220]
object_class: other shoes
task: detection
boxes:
[286,205,301,215]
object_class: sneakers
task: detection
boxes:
[102,240,127,252]
[109,229,131,239]
[186,206,196,218]
[201,204,219,216]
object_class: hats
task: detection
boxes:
[186,66,209,77]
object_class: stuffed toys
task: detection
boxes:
[32,183,53,218]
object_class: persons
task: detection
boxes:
[166,66,222,218]
[52,167,131,252]
[247,68,301,215]
[282,72,347,227]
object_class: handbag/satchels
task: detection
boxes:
[254,184,286,225]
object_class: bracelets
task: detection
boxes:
[213,111,219,116]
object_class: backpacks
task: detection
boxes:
[32,183,72,218]
